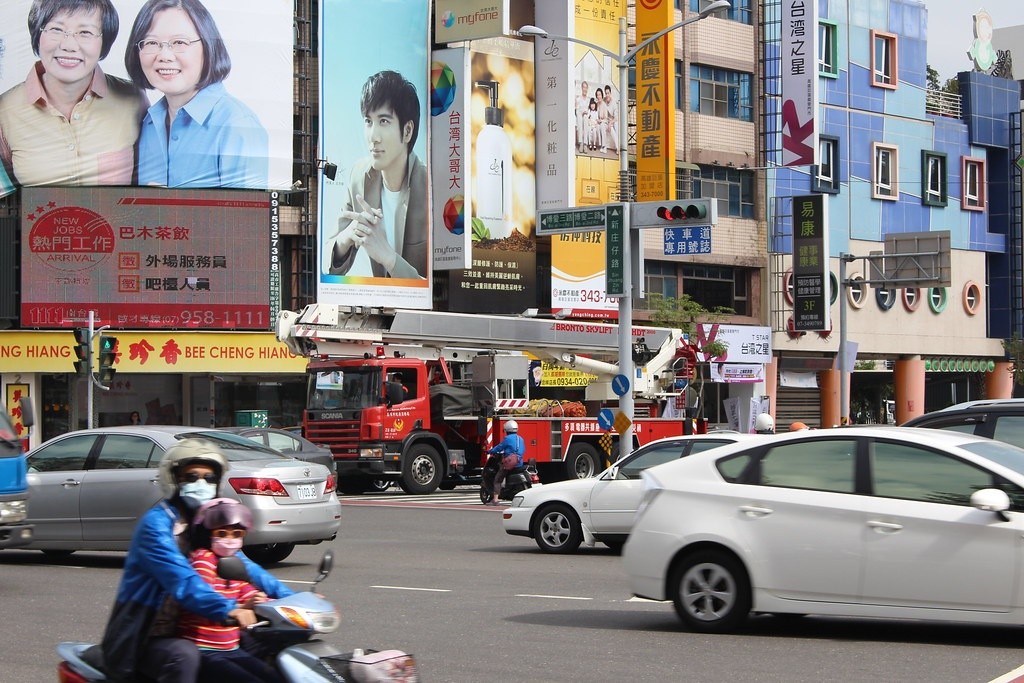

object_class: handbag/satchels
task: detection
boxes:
[502,452,519,471]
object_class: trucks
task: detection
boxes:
[0,396,35,551]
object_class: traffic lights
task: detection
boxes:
[98,335,117,382]
[73,329,89,375]
[656,204,705,221]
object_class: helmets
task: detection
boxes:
[754,413,774,431]
[502,420,519,433]
[786,421,810,432]
[157,438,230,503]
[191,497,255,532]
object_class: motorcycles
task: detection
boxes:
[55,551,421,683]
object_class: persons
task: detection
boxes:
[576,81,619,153]
[392,373,410,402]
[102,437,324,683]
[0,0,268,189]
[484,420,525,505]
[323,69,428,280]
[755,414,774,434]
[790,422,811,431]
[176,495,281,683]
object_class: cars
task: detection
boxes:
[25,425,342,564]
[504,430,768,554]
[621,424,1024,635]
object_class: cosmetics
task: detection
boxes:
[474,78,513,239]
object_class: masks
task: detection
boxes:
[177,480,219,511]
[209,536,244,557]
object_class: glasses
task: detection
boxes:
[176,472,220,483]
[134,36,202,55]
[209,528,247,539]
[39,26,104,42]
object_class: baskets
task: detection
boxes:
[319,648,421,683]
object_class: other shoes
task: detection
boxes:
[603,147,607,154]
[486,499,500,506]
[578,144,583,153]
[589,144,593,151]
[584,145,588,153]
[594,145,597,151]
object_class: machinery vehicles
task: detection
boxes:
[275,302,707,494]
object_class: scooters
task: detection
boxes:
[480,450,539,505]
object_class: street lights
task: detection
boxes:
[519,1,732,461]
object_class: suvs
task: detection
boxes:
[900,398,1024,450]
